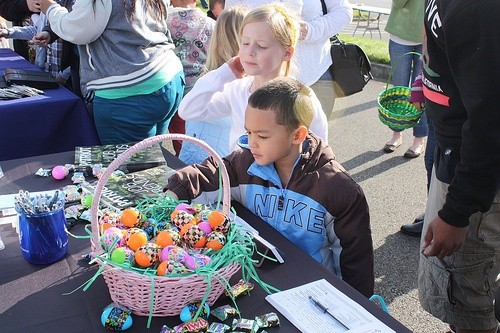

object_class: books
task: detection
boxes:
[82,164,178,215]
[74,142,167,176]
[265,278,396,333]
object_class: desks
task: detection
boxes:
[0,149,413,333]
[351,4,392,39]
[0,47,92,162]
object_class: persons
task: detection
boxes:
[384,0,500,333]
[0,0,354,166]
[162,76,375,300]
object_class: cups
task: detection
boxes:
[15,195,69,264]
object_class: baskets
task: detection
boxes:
[91,133,240,318]
[377,52,425,131]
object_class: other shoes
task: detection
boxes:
[404,147,424,159]
[383,141,403,153]
[401,212,425,237]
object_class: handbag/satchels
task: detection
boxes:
[320,0,373,98]
